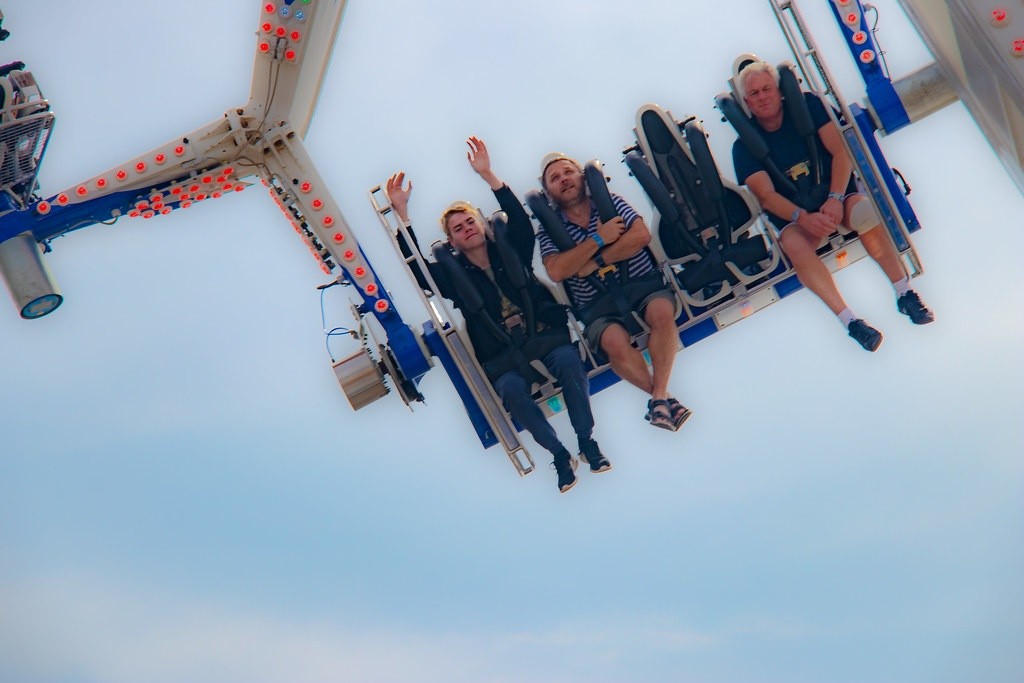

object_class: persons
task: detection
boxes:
[732,61,933,351]
[386,136,611,494]
[536,152,691,433]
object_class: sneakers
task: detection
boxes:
[897,289,934,325]
[847,318,883,352]
[550,446,579,493]
[577,439,612,474]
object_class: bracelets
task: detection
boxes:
[593,254,606,267]
[828,192,844,202]
[592,233,604,248]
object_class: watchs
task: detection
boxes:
[792,208,806,223]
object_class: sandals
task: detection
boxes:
[645,399,677,432]
[667,397,692,432]
